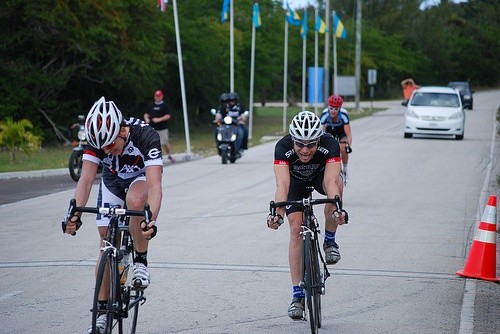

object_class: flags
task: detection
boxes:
[220,0,230,22]
[285,1,302,26]
[299,12,308,37]
[156,0,169,12]
[253,3,262,28]
[334,14,347,39]
[313,15,329,34]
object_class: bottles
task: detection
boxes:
[121,246,129,280]
[118,260,126,285]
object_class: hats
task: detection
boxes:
[155,90,162,97]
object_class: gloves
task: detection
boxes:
[344,145,352,154]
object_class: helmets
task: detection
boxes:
[220,94,229,103]
[289,111,324,141]
[85,96,123,150]
[227,93,237,107]
[327,94,343,108]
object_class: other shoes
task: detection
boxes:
[168,156,175,163]
[343,171,348,187]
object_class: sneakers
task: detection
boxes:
[88,314,108,334]
[130,262,150,288]
[323,239,341,265]
[288,293,306,319]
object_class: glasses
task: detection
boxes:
[104,137,119,150]
[293,138,319,149]
[329,107,339,110]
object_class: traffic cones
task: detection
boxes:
[455,194,499,282]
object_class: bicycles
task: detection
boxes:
[63,188,154,334]
[269,195,342,334]
[335,136,352,187]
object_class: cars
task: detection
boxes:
[450,81,475,111]
[401,86,465,140]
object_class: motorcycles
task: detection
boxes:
[209,108,250,164]
[67,114,106,182]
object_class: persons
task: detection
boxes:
[215,93,249,149]
[62,97,163,334]
[267,111,348,318]
[401,78,420,99]
[319,94,352,164]
[144,90,175,162]
